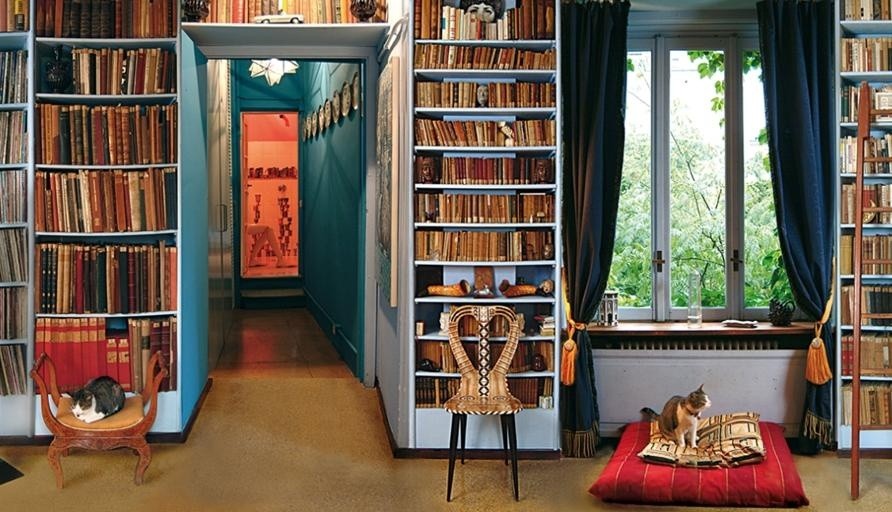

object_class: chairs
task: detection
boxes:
[443,302,522,505]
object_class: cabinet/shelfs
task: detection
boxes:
[404,0,563,461]
[2,3,178,420]
[833,0,892,458]
[246,165,298,271]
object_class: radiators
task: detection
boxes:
[589,341,815,438]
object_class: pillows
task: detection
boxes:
[636,409,766,467]
[587,421,810,508]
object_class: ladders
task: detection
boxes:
[851,79,892,502]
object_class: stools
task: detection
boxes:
[30,350,168,489]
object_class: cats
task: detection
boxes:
[65,375,126,424]
[641,382,713,449]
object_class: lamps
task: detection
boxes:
[247,58,299,86]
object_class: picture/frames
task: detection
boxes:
[372,56,398,308]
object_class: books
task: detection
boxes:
[838,0,890,429]
[0,288,27,340]
[414,317,556,408]
[0,344,27,397]
[176,0,389,26]
[414,192,554,263]
[413,119,556,186]
[0,228,29,284]
[34,168,178,232]
[37,45,177,95]
[0,49,28,104]
[0,0,178,38]
[413,1,556,40]
[413,43,556,108]
[34,104,176,165]
[36,240,177,314]
[34,319,178,394]
[1,111,28,164]
[0,170,25,224]
